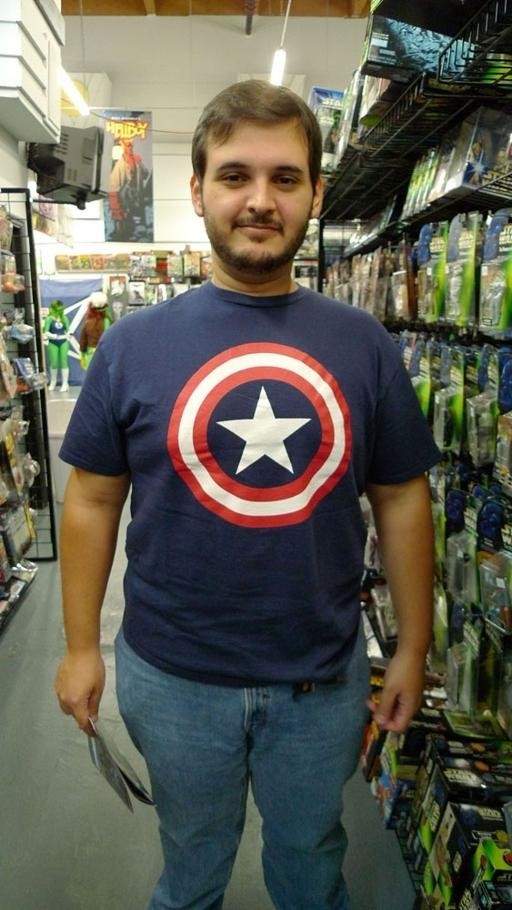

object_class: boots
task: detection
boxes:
[48,368,69,392]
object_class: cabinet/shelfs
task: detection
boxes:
[1,187,56,633]
[316,1,511,910]
[56,255,320,317]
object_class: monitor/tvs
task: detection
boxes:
[27,126,114,202]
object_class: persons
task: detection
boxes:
[44,301,70,392]
[79,293,111,370]
[53,81,447,910]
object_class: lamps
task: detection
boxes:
[270,49,287,86]
[59,66,91,118]
